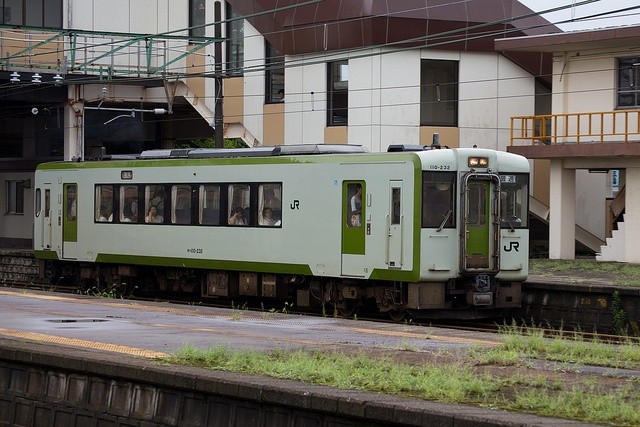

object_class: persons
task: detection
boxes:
[229,209,246,225]
[264,208,286,226]
[123,208,133,222]
[145,207,164,223]
[350,184,361,225]
[99,204,109,222]
[268,190,282,212]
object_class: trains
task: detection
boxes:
[30,146,530,320]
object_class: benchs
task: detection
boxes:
[263,208,281,225]
[202,208,220,225]
[176,208,191,223]
